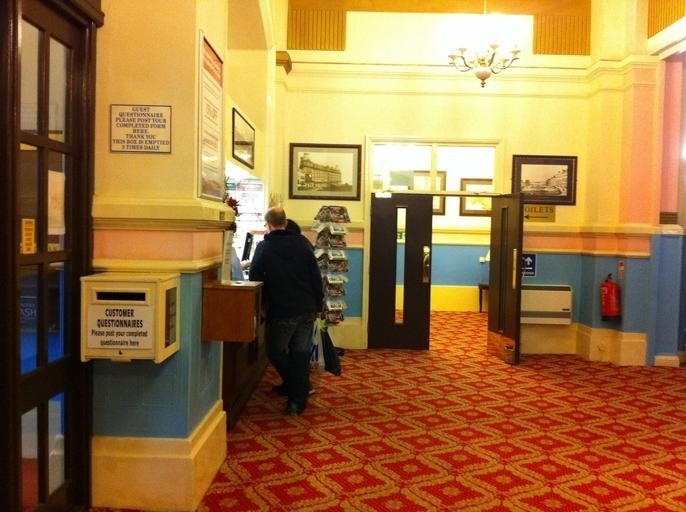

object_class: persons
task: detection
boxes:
[250,204,325,415]
[271,219,316,398]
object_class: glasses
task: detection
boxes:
[263,222,269,227]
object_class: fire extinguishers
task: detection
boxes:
[600,273,621,322]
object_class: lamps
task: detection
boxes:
[446,1,526,88]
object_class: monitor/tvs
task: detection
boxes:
[242,232,253,261]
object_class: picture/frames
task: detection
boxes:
[512,156,577,206]
[288,143,362,201]
[406,170,494,215]
[231,107,256,170]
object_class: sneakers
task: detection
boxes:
[273,381,316,412]
